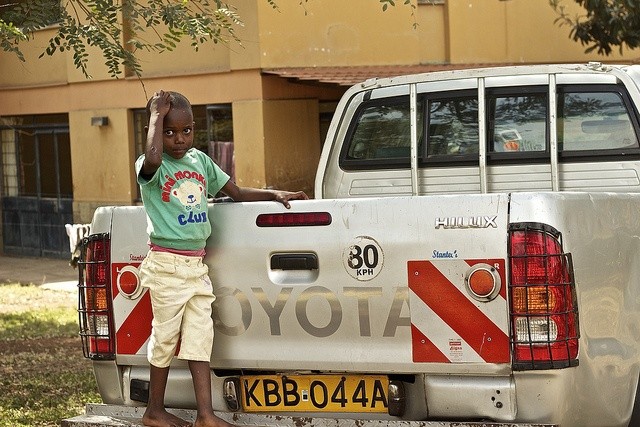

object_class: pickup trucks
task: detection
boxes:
[69,62,639,427]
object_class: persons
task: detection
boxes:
[135,90,310,426]
